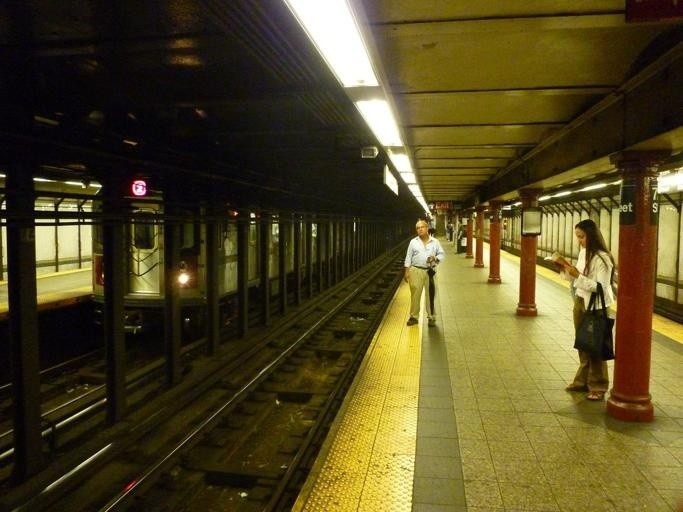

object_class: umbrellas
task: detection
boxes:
[425,256,435,321]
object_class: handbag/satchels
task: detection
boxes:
[572,282,615,361]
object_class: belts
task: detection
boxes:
[414,265,436,269]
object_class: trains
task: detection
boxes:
[87,182,414,343]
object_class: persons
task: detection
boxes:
[401,219,445,328]
[427,219,433,237]
[447,224,453,241]
[453,220,463,255]
[556,218,615,401]
[446,222,450,242]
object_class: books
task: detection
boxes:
[542,250,570,273]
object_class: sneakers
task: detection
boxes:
[566,384,604,401]
[406,319,436,327]
